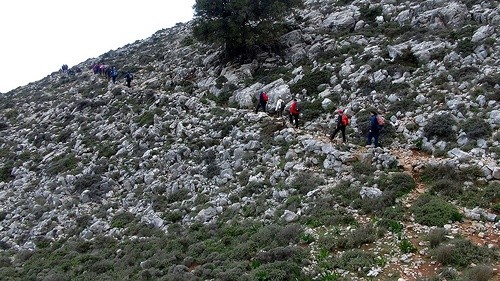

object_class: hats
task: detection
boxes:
[338,109,343,113]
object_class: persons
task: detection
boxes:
[275,96,283,117]
[65,64,68,70]
[62,64,65,73]
[93,63,117,84]
[366,110,381,148]
[335,109,346,142]
[124,71,133,88]
[255,92,266,114]
[289,99,300,129]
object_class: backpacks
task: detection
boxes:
[342,114,348,126]
[263,93,268,101]
[296,103,301,112]
[375,115,384,125]
[129,73,133,80]
[279,100,285,110]
